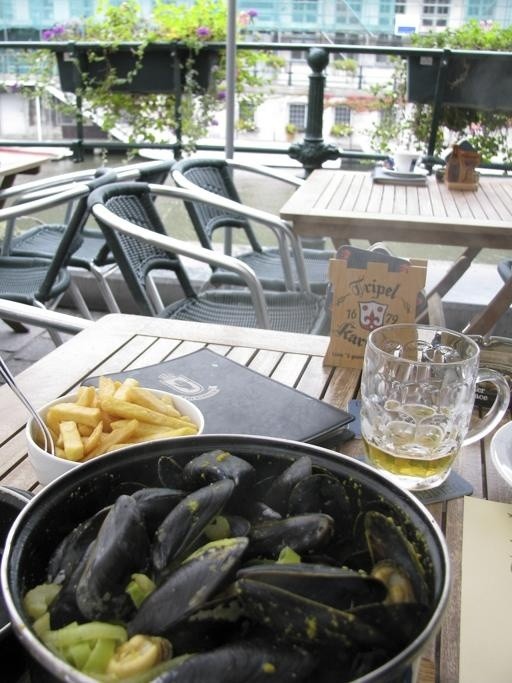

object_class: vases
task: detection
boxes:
[50,41,216,93]
[397,44,511,115]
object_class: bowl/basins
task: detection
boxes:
[26,386,204,492]
[0,432,453,683]
[1,483,44,644]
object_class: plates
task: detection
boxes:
[489,419,512,487]
[383,167,428,177]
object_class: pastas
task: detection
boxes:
[26,579,145,680]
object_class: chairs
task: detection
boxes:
[9,156,171,311]
[87,181,328,336]
[168,158,336,293]
[2,169,115,347]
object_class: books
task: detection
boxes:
[372,165,426,185]
[80,346,356,449]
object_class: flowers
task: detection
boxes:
[364,16,512,174]
[0,0,290,162]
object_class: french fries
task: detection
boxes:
[39,375,199,466]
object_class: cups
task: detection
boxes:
[393,151,418,172]
[360,323,509,490]
